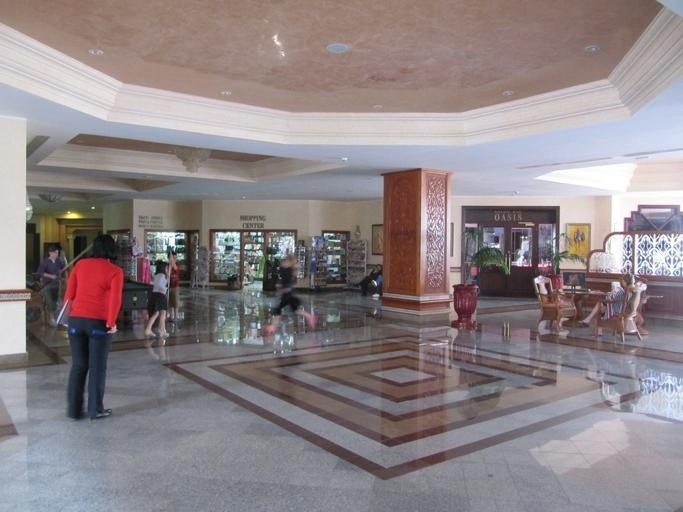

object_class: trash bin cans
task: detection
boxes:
[228,276,241,290]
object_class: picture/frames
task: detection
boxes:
[558,269,588,292]
[372,224,384,255]
[566,223,591,257]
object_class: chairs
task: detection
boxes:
[595,281,647,343]
[533,275,577,333]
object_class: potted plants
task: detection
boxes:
[546,233,586,294]
[451,227,511,329]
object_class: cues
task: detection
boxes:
[165,249,172,323]
[37,241,93,291]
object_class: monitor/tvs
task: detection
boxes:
[562,272,585,289]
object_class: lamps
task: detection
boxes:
[26,188,33,221]
[175,147,211,173]
[38,191,63,202]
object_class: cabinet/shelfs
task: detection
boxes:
[211,234,294,282]
[310,236,329,290]
[346,240,367,290]
[324,238,346,274]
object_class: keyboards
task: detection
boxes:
[563,289,586,291]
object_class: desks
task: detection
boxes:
[117,280,153,329]
[553,292,649,336]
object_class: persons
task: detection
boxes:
[141,338,172,416]
[164,250,180,323]
[260,253,316,337]
[142,262,170,337]
[35,246,71,326]
[367,263,383,320]
[574,273,635,327]
[57,232,125,421]
[588,333,641,413]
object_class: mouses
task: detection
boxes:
[588,288,591,292]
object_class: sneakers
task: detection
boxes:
[372,293,380,298]
[145,327,169,337]
[578,320,589,327]
[168,316,178,322]
[91,408,111,420]
[371,279,377,288]
[353,283,359,288]
[361,292,366,296]
[49,319,57,327]
